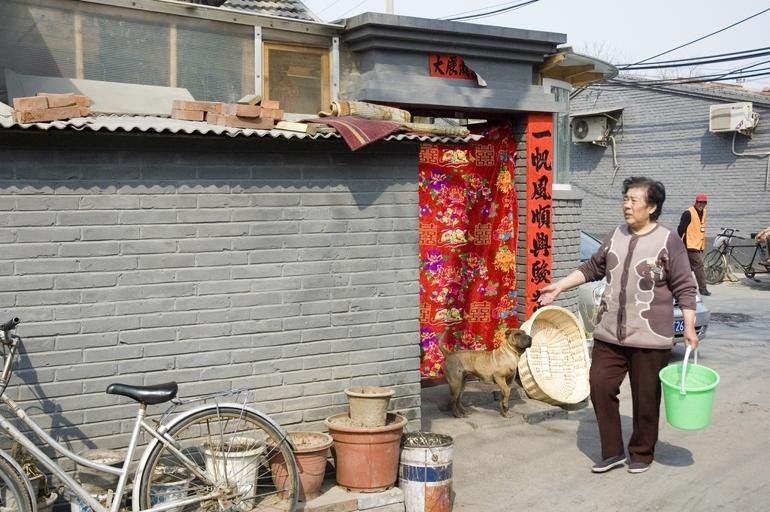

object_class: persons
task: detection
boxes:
[677,193,712,297]
[537,174,699,474]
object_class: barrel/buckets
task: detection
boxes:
[397,411,455,512]
[659,343,721,432]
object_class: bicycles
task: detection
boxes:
[703,227,770,284]
[0,316,300,512]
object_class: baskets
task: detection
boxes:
[517,304,591,408]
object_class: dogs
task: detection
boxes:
[439,326,533,418]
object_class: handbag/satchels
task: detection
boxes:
[714,237,731,248]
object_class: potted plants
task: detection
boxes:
[6,438,50,508]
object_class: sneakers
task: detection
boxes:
[700,289,712,297]
[591,455,627,474]
[628,461,651,473]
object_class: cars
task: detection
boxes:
[579,230,710,346]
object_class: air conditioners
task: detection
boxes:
[708,102,754,135]
[571,117,609,148]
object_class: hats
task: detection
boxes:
[697,194,708,203]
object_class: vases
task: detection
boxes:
[149,466,196,512]
[0,492,58,512]
[200,436,265,510]
[71,449,126,512]
[264,385,456,512]
